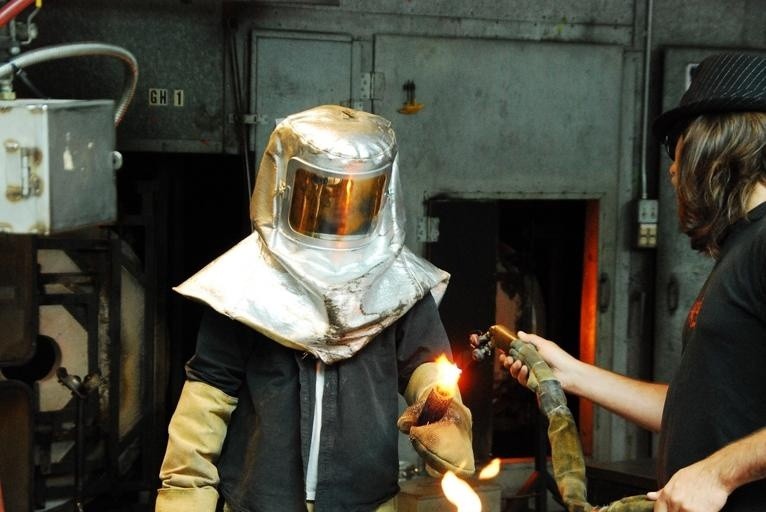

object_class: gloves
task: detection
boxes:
[397,361,475,480]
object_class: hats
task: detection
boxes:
[651,54,765,144]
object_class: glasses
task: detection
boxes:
[664,115,701,161]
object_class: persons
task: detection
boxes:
[154,104,476,512]
[497,50,766,512]
